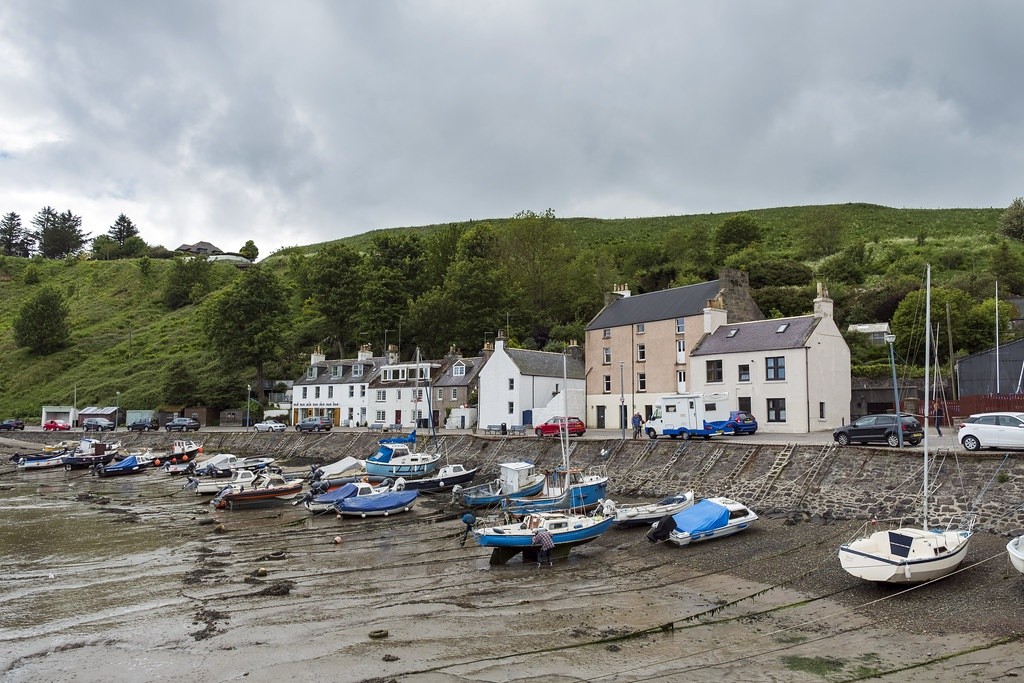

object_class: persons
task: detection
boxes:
[631,412,644,439]
[929,403,944,437]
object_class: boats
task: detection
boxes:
[332,487,419,519]
[7,435,609,522]
[589,489,695,530]
[1005,533,1024,576]
[644,495,760,549]
[447,461,547,510]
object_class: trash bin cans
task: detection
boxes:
[416,419,422,427]
[501,423,507,434]
[421,419,428,428]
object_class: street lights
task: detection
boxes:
[114,392,120,431]
[246,384,252,432]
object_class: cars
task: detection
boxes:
[42,419,70,431]
[0,419,25,432]
[535,415,586,438]
[730,410,758,436]
[252,419,287,433]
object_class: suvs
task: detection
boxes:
[832,413,924,447]
[164,417,201,433]
[957,411,1024,452]
[82,417,115,432]
[295,416,333,432]
[126,417,160,432]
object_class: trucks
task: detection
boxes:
[644,391,731,440]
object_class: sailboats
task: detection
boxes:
[363,346,442,483]
[836,261,978,587]
[462,349,615,567]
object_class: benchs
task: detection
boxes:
[484,425,502,435]
[508,425,527,435]
[390,424,403,432]
[368,424,383,431]
